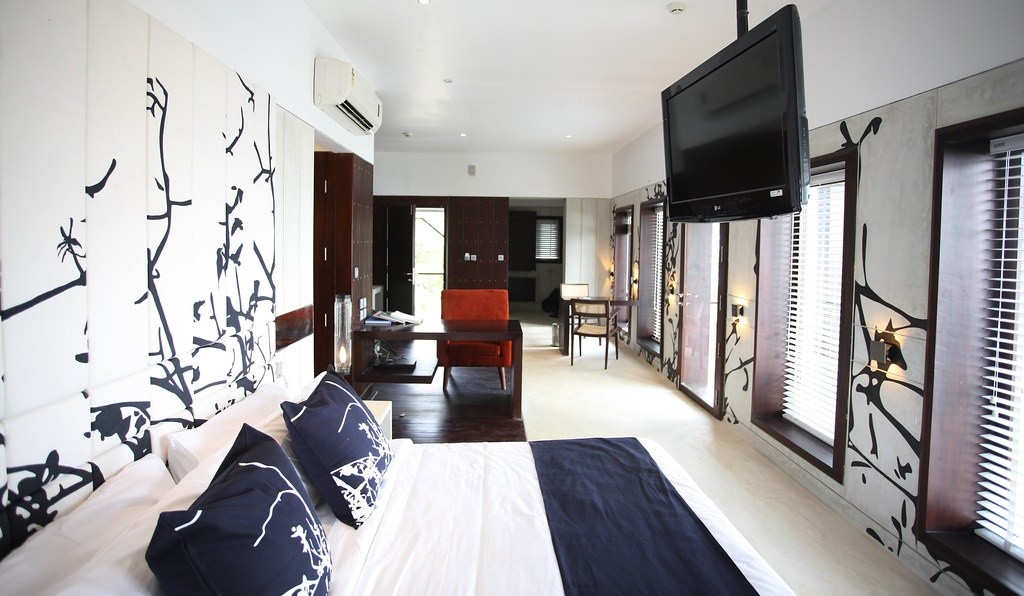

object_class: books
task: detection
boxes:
[365,311,422,324]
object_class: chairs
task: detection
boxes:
[561,284,601,345]
[437,289,512,392]
[571,297,619,369]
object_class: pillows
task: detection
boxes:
[281,364,394,527]
[145,423,332,596]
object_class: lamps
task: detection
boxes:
[333,295,353,375]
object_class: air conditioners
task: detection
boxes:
[314,57,383,135]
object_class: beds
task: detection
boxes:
[0,369,800,596]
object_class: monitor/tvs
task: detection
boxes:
[660,4,811,224]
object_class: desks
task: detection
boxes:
[350,319,524,420]
[559,296,639,356]
